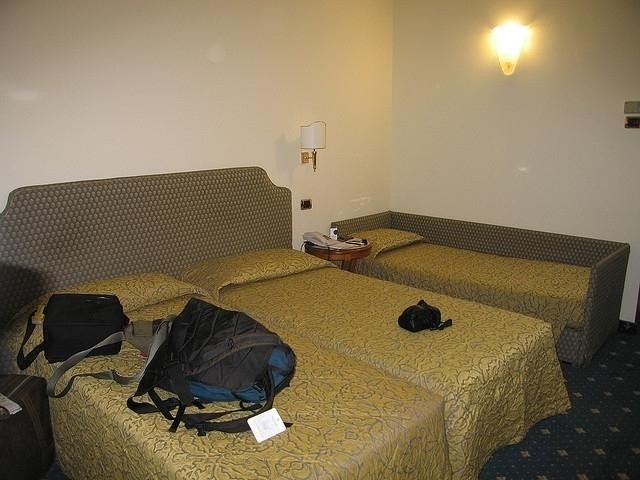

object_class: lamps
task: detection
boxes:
[300,121,326,172]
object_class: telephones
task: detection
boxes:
[303,232,333,247]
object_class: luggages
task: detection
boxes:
[1,374,55,480]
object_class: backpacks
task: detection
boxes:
[135,297,297,403]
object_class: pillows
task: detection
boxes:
[0,272,215,380]
[175,247,338,303]
[339,228,424,275]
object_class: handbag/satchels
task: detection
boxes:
[398,299,452,333]
[43,294,130,364]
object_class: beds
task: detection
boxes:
[0,294,451,480]
[220,266,573,480]
[330,209,630,368]
